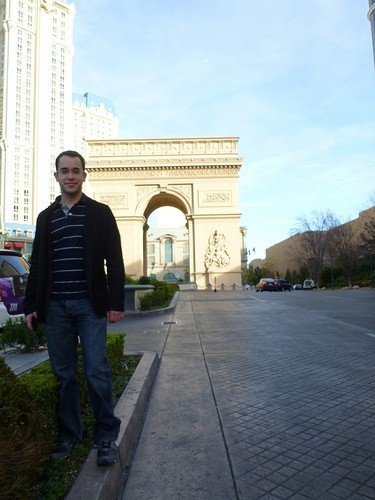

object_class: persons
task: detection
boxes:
[23,150,127,470]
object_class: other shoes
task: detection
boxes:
[97,439,120,466]
[47,439,78,459]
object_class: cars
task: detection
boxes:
[274,279,292,291]
[256,278,278,293]
[293,284,302,290]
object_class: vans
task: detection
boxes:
[0,248,35,332]
[303,279,314,289]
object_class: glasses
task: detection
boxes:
[56,168,85,178]
